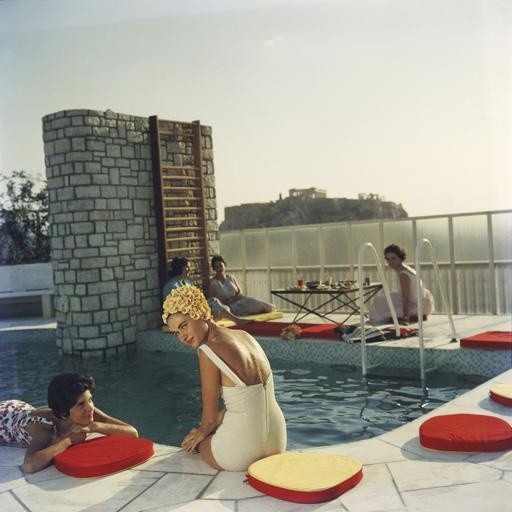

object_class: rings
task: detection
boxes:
[193,440,194,443]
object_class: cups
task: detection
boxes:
[364,277,370,286]
[297,279,304,287]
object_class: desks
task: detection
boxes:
[271,282,382,325]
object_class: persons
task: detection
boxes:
[369,243,435,325]
[161,284,287,471]
[207,255,275,316]
[0,371,139,474]
[162,257,255,326]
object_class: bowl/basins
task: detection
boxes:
[306,281,320,288]
